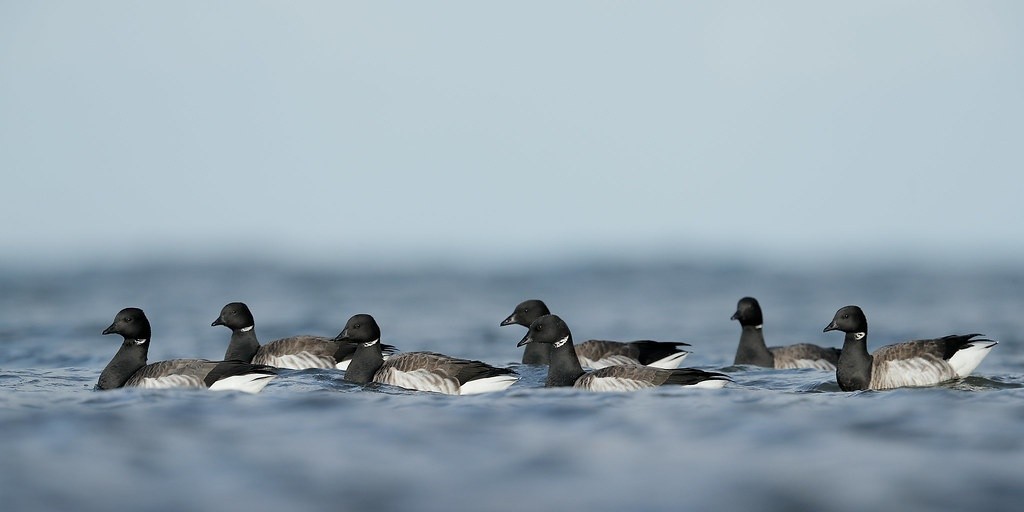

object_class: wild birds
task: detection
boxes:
[501,299,694,370]
[92,307,419,394]
[824,306,998,393]
[517,314,735,389]
[729,296,844,370]
[210,301,399,373]
[334,314,521,395]
